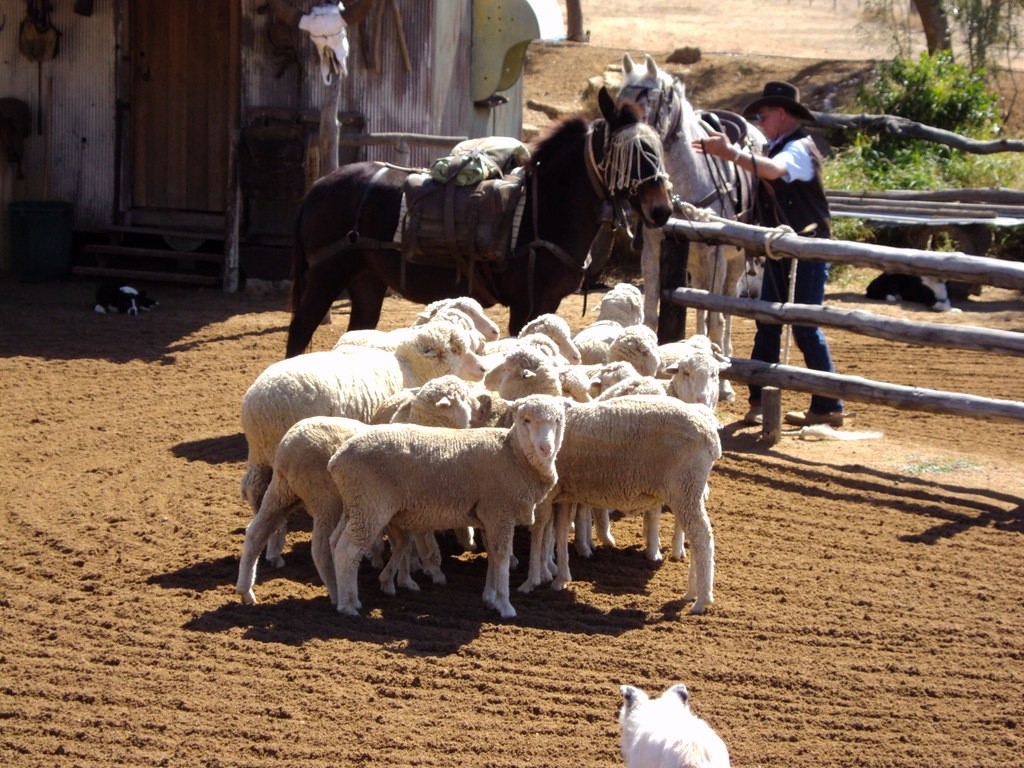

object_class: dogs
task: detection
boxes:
[618,681,731,768]
[93,285,159,317]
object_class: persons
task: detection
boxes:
[692,80,844,427]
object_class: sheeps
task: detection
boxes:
[236,280,731,618]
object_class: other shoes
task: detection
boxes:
[784,407,843,427]
[745,404,763,424]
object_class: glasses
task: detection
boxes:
[756,108,780,122]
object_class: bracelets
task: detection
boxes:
[734,150,741,163]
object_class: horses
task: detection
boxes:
[283,52,771,402]
[864,271,988,313]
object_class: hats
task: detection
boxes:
[743,81,816,122]
